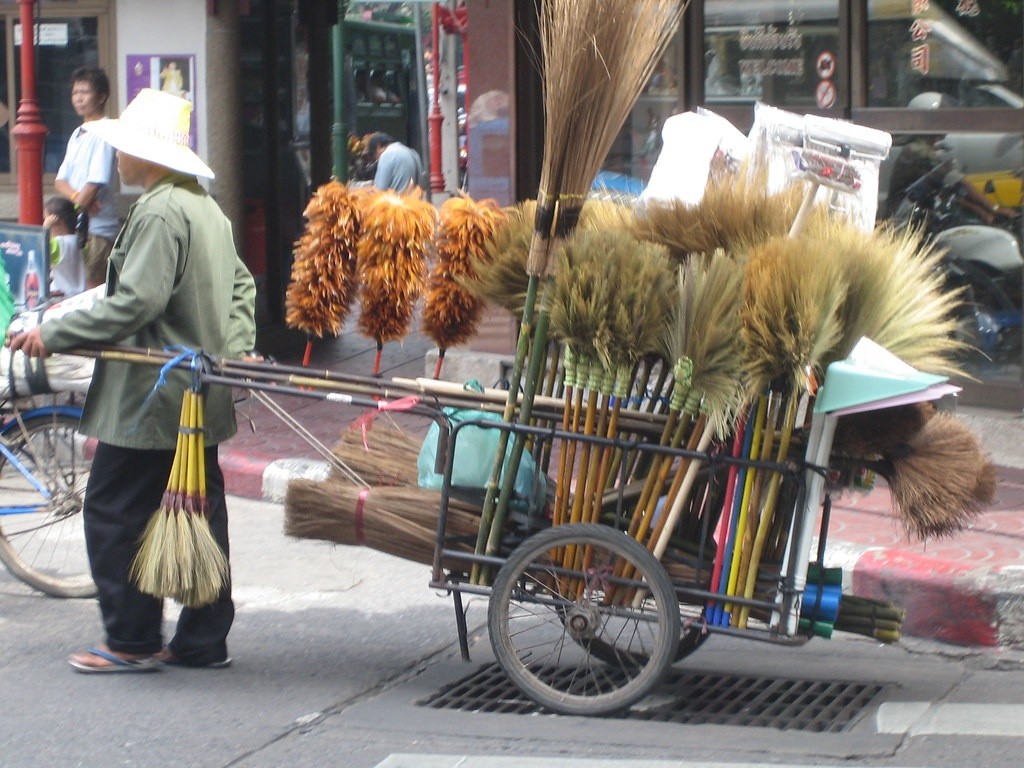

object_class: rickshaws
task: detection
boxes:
[8,330,832,714]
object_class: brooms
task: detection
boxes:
[125,387,229,608]
[469,0,999,631]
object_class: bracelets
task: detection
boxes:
[992,204,1000,211]
[71,191,79,201]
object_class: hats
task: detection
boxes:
[87,88,216,180]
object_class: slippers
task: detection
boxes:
[67,647,161,673]
[153,645,232,668]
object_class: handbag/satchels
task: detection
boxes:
[417,379,547,518]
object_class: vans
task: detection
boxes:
[631,0,1024,220]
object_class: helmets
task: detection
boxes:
[907,91,953,110]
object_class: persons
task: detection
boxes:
[9,88,276,674]
[882,91,1017,226]
[161,62,182,92]
[367,132,423,195]
[42,65,122,299]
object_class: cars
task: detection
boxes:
[469,91,648,208]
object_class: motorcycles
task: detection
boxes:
[932,179,1024,370]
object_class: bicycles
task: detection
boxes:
[0,391,117,598]
